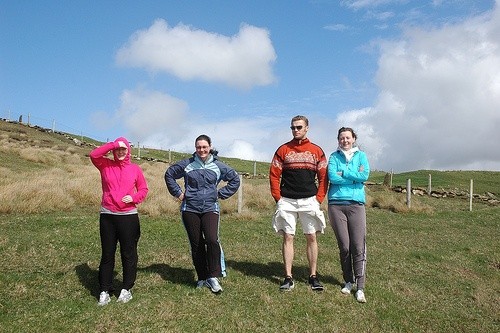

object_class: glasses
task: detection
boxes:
[290,126,306,130]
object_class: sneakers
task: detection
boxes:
[279,275,294,292]
[116,289,132,304]
[97,291,111,307]
[195,280,205,291]
[205,277,223,293]
[308,274,323,290]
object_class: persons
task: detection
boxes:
[89,137,148,305]
[327,127,369,302]
[165,135,240,292]
[269,115,329,290]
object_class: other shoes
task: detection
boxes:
[355,290,366,303]
[341,281,355,294]
